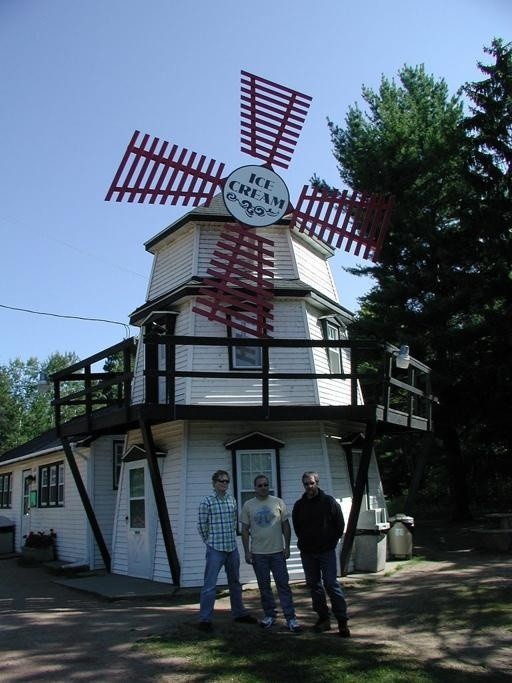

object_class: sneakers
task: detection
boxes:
[287,620,304,632]
[261,616,277,628]
[198,622,212,631]
[235,615,257,624]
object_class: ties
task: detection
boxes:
[257,484,269,488]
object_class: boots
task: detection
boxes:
[309,606,330,632]
[338,618,350,638]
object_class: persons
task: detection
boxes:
[292,471,350,637]
[198,470,258,633]
[238,475,302,633]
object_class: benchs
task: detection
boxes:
[456,525,490,545]
[471,528,512,553]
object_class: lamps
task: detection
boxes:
[25,475,35,486]
[25,474,36,485]
[391,345,411,370]
[70,442,77,451]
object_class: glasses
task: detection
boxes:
[304,482,314,486]
[215,479,229,483]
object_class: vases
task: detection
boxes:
[21,546,54,567]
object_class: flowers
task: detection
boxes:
[22,529,57,549]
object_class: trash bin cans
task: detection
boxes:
[387,513,414,560]
[354,507,390,572]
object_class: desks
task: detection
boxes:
[484,512,512,528]
